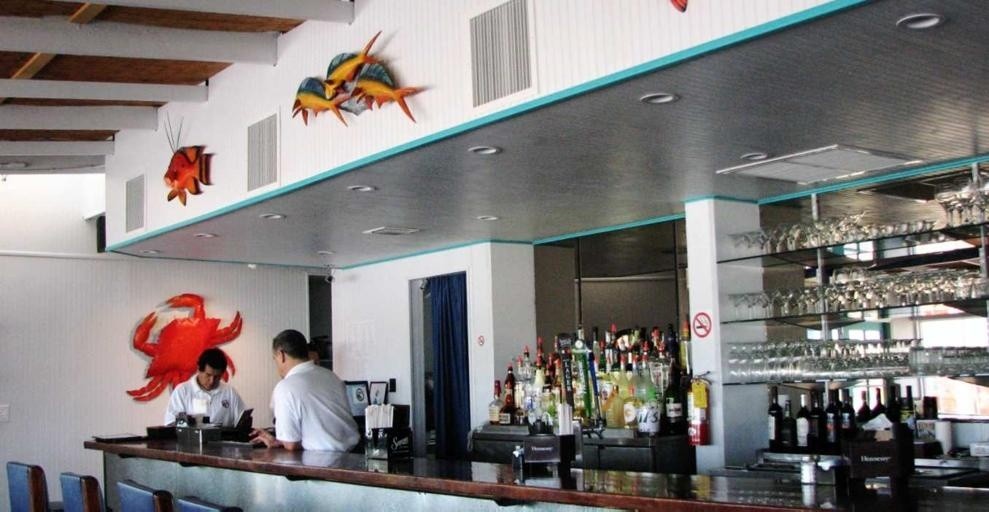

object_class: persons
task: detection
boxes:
[249,330,366,456]
[165,348,247,428]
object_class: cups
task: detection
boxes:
[923,396,939,419]
[798,455,816,485]
[800,483,815,507]
[808,220,930,247]
[908,345,989,375]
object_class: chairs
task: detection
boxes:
[115,477,175,511]
[58,471,106,511]
[4,461,50,512]
[174,494,245,512]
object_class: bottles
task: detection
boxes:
[581,470,710,501]
[489,322,691,431]
[760,381,917,452]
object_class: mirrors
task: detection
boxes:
[528,211,692,373]
[757,152,989,428]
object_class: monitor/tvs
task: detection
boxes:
[344,380,371,420]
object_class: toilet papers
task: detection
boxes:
[935,420,953,456]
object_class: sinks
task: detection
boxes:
[939,472,989,492]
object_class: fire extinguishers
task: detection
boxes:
[687,371,712,445]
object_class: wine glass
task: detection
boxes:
[728,338,921,379]
[932,171,989,229]
[723,208,864,258]
[725,266,984,321]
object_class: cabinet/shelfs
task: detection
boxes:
[470,422,695,474]
[714,217,989,388]
